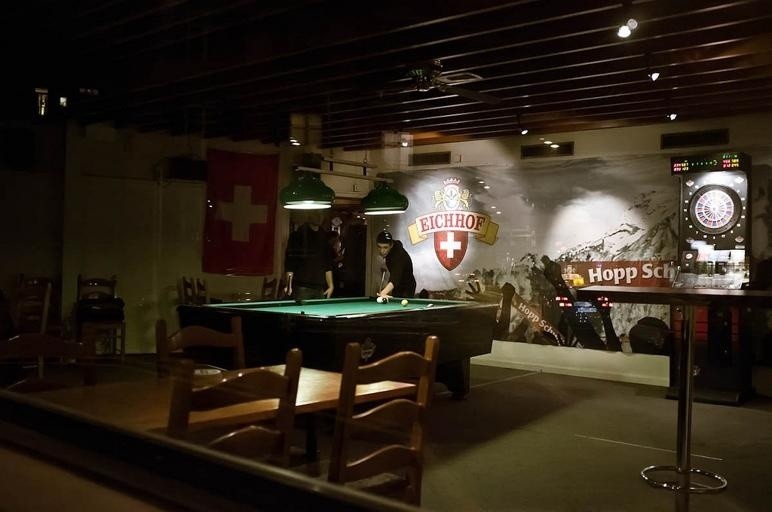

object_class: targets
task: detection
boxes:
[689,184,742,235]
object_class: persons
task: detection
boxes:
[326,231,339,246]
[465,261,670,355]
[284,210,335,300]
[376,232,416,302]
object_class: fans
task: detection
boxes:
[360,41,503,107]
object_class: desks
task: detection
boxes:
[571,282,771,496]
[176,295,499,402]
[31,360,419,456]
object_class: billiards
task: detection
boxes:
[376,297,383,303]
[382,298,387,304]
[401,299,408,306]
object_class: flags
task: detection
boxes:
[202,149,280,276]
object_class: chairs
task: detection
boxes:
[150,315,248,370]
[167,348,304,473]
[284,333,440,506]
[0,271,128,380]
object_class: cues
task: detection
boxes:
[379,271,385,292]
[284,274,291,298]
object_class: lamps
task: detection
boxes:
[279,114,410,217]
